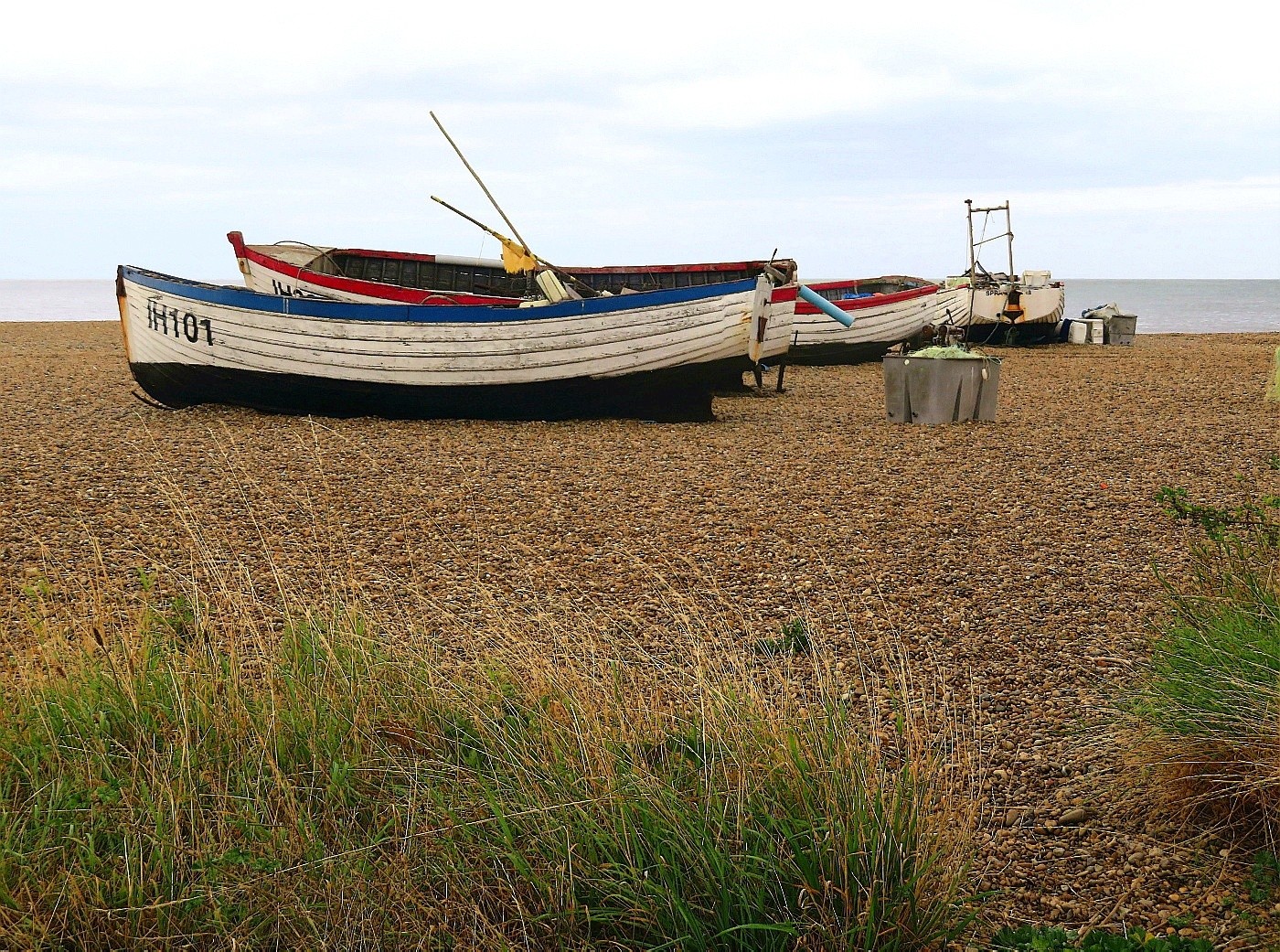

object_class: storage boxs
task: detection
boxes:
[1023,269,1051,286]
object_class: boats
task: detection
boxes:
[224,110,1069,367]
[114,246,775,411]
